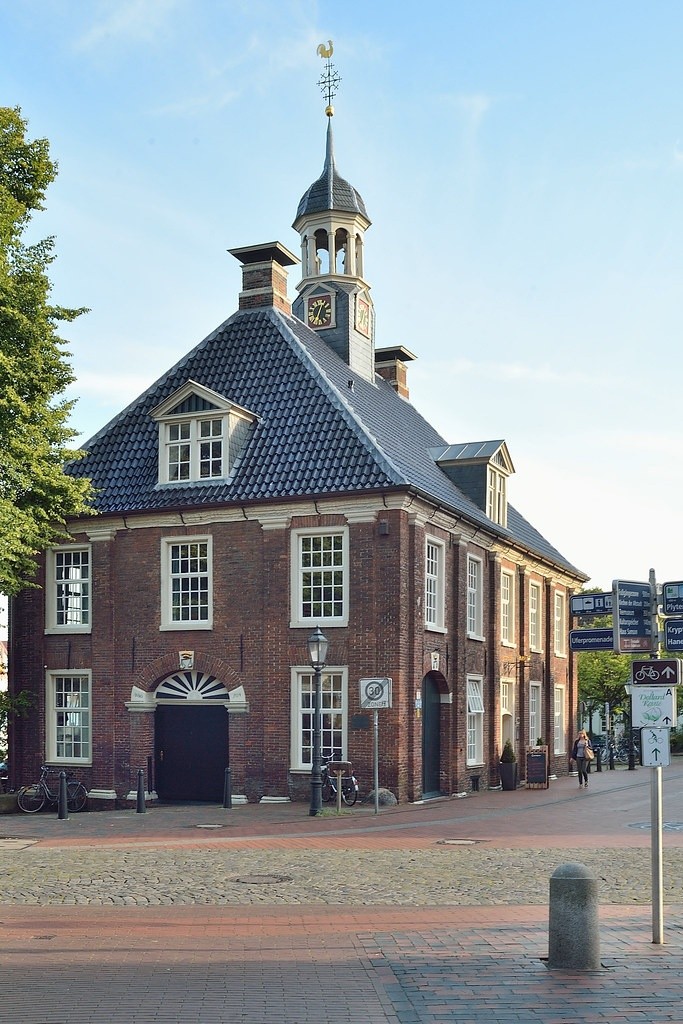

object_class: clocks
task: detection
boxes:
[302,292,335,330]
[355,296,371,338]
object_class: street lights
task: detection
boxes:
[308,626,330,817]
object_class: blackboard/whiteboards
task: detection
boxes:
[527,754,546,783]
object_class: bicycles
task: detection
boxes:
[598,741,637,763]
[320,753,359,807]
[17,766,88,814]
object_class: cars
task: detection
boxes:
[590,736,608,755]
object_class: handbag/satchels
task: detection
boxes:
[583,741,594,761]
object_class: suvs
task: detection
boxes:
[620,728,640,748]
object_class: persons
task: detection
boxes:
[570,730,593,789]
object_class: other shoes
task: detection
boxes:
[579,785,582,789]
[585,781,588,787]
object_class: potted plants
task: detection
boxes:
[499,741,518,792]
